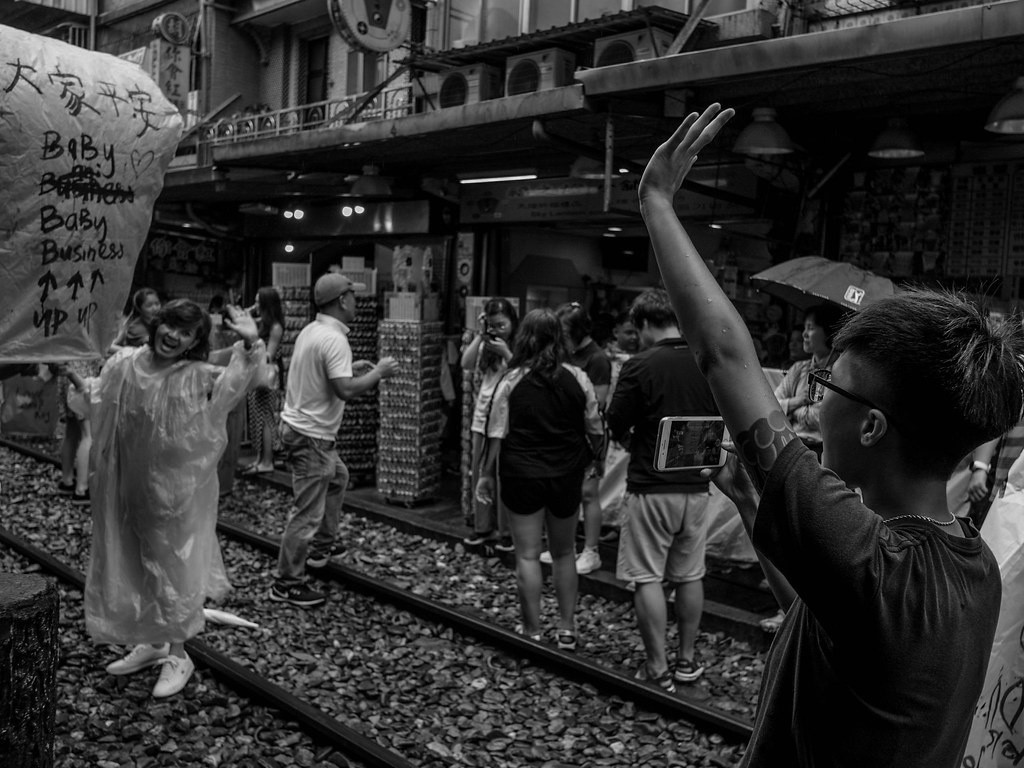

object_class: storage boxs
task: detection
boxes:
[272,262,312,288]
[389,292,440,321]
[464,295,520,331]
[333,268,378,297]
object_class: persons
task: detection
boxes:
[56,298,266,696]
[270,273,400,604]
[462,289,843,693]
[55,287,285,504]
[637,103,1024,768]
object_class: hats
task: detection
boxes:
[314,274,368,305]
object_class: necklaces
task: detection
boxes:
[883,511,956,525]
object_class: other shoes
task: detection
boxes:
[761,609,788,633]
[243,464,274,474]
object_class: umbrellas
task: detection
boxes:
[749,256,902,314]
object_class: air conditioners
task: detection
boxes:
[504,48,576,97]
[435,63,503,110]
[594,26,674,67]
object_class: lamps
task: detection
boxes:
[983,76,1024,135]
[867,117,927,160]
[568,134,621,180]
[731,107,794,156]
[349,164,392,196]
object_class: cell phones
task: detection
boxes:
[651,415,733,471]
[480,333,496,342]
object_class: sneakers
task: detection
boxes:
[56,477,77,494]
[105,643,171,676]
[308,545,348,567]
[538,551,555,563]
[495,535,519,552]
[153,649,193,696]
[464,532,499,544]
[574,548,602,574]
[71,488,92,505]
[270,585,326,605]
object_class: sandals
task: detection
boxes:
[673,658,705,680]
[623,665,675,692]
[553,627,578,648]
[513,625,545,640]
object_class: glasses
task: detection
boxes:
[808,369,885,422]
